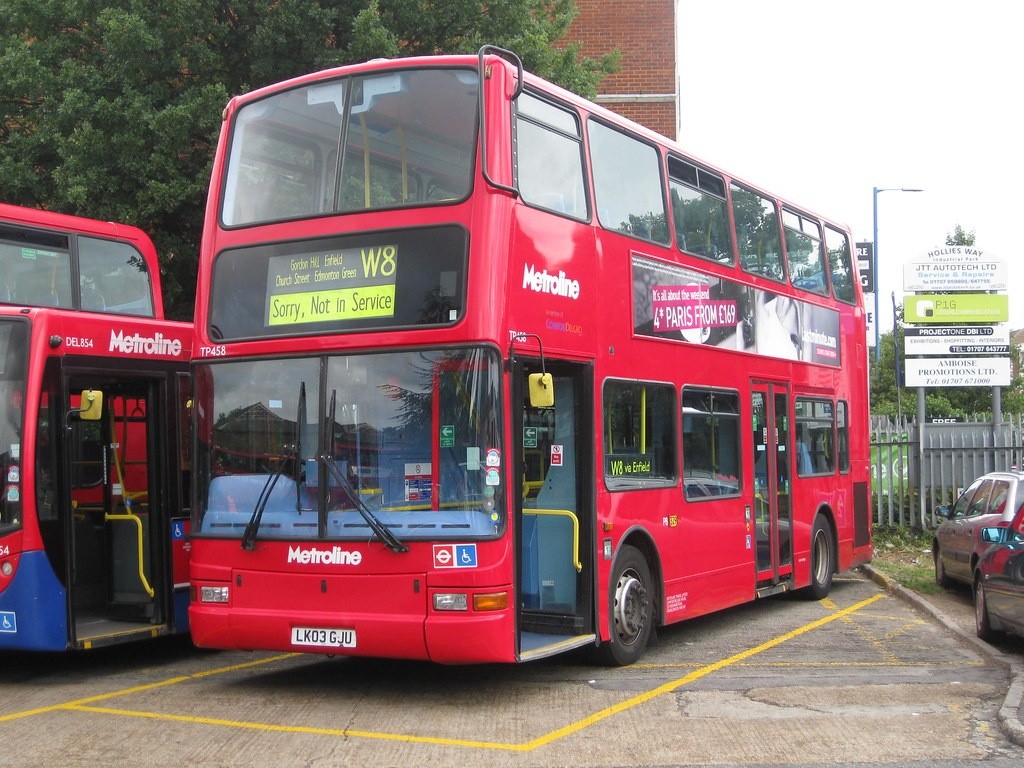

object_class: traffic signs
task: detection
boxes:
[903,245,1010,386]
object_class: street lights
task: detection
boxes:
[873,186,925,364]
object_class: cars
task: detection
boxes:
[932,471,1024,606]
[974,501,1024,641]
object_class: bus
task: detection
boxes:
[187,47,871,668]
[0,202,196,653]
